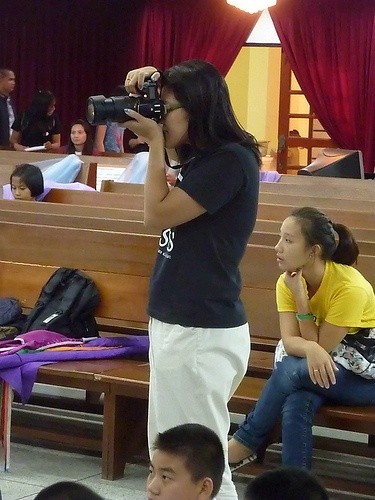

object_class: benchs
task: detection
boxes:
[0,150,375,500]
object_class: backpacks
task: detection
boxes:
[20,268,98,338]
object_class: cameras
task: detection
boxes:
[85,78,165,126]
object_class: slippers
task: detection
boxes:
[228,452,256,473]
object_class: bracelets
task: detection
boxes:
[297,313,314,320]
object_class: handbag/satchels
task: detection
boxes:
[274,327,375,379]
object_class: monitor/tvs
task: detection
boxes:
[297,148,364,180]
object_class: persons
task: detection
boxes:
[228,208,375,471]
[10,163,44,201]
[117,58,261,500]
[147,423,225,500]
[243,470,331,500]
[0,70,161,158]
[34,481,104,500]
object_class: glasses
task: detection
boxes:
[164,101,182,114]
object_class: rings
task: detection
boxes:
[314,370,318,372]
[127,79,131,80]
